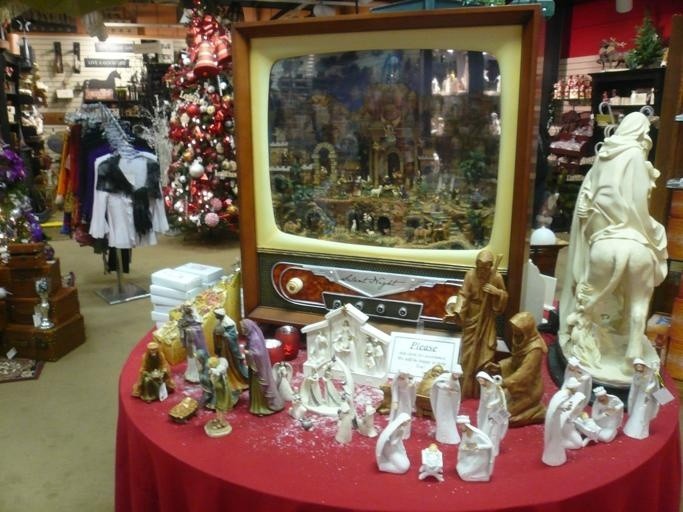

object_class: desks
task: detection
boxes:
[114,325,683,512]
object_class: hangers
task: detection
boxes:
[65,103,101,124]
[101,113,138,159]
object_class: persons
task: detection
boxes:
[132,110,670,483]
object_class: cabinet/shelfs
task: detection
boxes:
[587,68,665,166]
